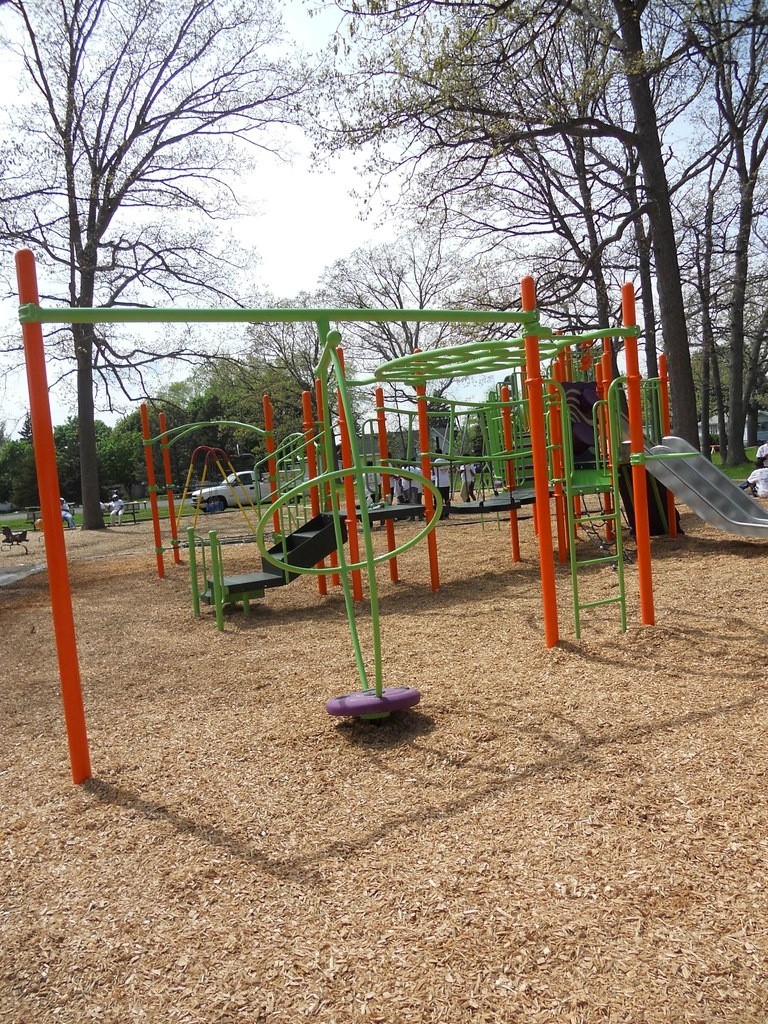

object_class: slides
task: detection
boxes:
[622,436,768,535]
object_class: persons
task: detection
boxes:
[108,493,125,526]
[174,484,180,498]
[100,502,105,516]
[738,459,768,499]
[59,497,76,530]
[755,440,768,467]
[363,450,476,527]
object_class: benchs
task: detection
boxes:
[25,502,75,531]
[102,502,140,524]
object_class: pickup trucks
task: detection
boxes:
[191,471,304,514]
